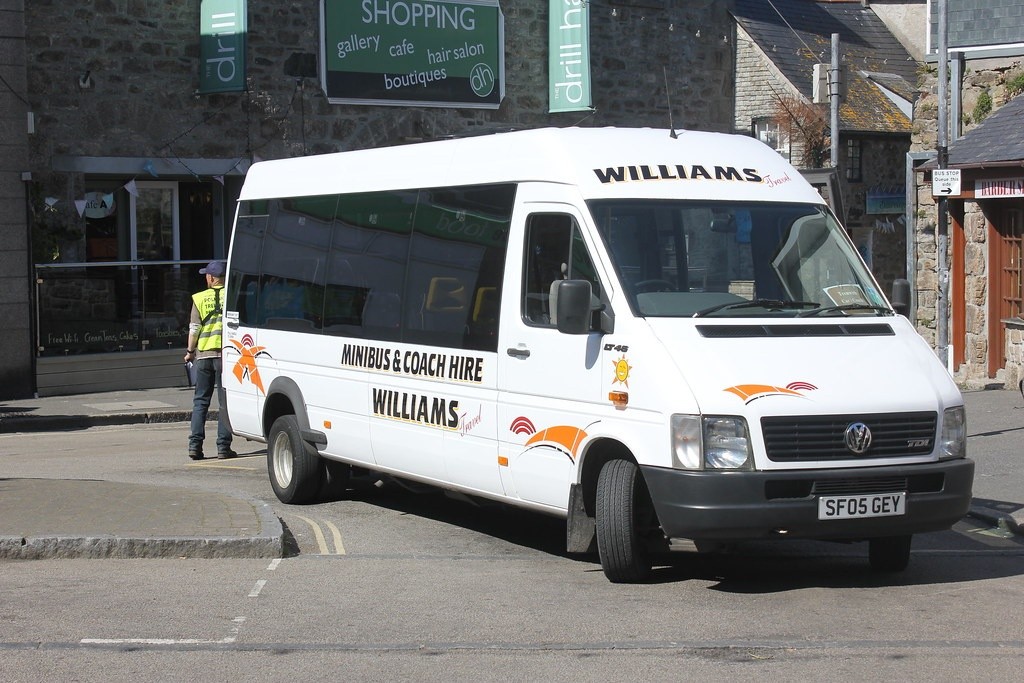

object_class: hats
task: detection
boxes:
[199,260,226,277]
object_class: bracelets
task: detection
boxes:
[187,350,195,353]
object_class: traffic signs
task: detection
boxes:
[931,168,961,196]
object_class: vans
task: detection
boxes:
[221,126,976,583]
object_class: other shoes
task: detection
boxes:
[189,450,204,458]
[218,450,237,459]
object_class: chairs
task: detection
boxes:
[361,278,500,337]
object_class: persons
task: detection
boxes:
[183,261,239,460]
